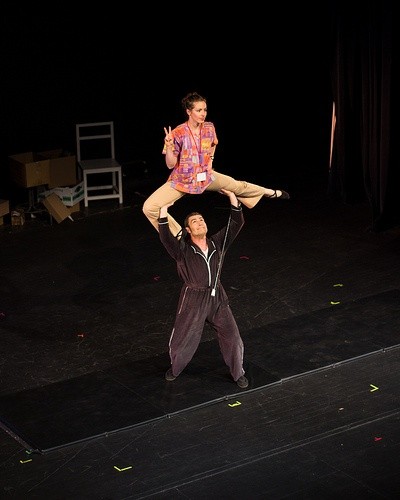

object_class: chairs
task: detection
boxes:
[75,122,123,207]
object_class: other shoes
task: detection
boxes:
[175,227,187,252]
[237,376,249,387]
[166,368,176,381]
[266,189,290,200]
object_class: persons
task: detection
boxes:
[157,188,250,388]
[142,93,291,253]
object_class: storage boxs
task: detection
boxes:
[0,150,85,226]
